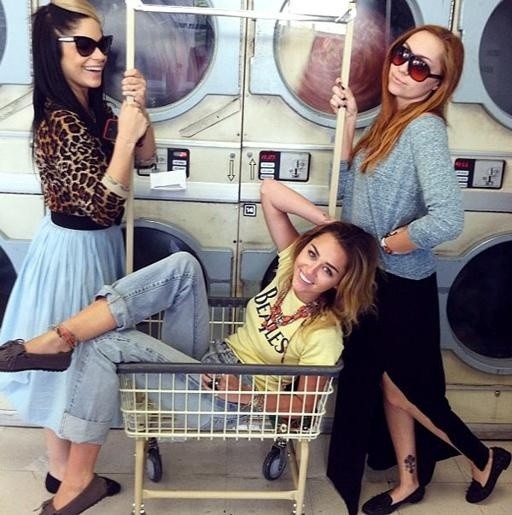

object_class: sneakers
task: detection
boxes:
[362,480,424,514]
[0,340,74,372]
[33,469,122,514]
[465,446,511,504]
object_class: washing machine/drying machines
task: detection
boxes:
[445,3,510,214]
[112,198,240,344]
[35,3,243,205]
[0,193,43,330]
[426,211,510,441]
[247,3,453,213]
[0,0,33,183]
[239,201,345,345]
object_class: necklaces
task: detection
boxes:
[257,278,317,332]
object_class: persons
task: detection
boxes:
[0,0,161,499]
[0,177,382,515]
[326,22,512,515]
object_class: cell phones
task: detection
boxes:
[103,119,147,147]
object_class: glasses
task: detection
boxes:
[391,45,444,83]
[58,34,114,57]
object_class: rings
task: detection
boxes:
[209,381,216,390]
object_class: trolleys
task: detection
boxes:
[115,0,360,515]
